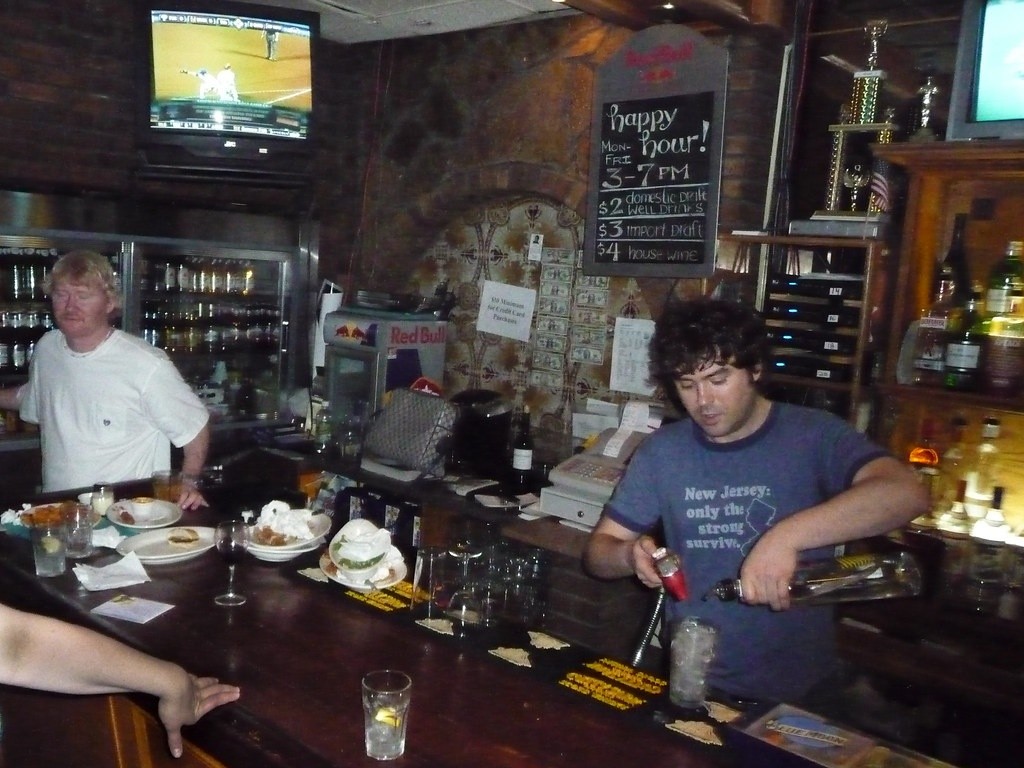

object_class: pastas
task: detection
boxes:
[23,505,62,527]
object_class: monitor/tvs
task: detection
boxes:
[131,0,322,174]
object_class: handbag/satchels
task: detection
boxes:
[358,386,458,480]
[312,474,422,574]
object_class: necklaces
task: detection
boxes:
[63,328,115,358]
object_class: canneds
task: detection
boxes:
[164,259,256,295]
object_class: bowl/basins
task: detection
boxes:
[79,492,114,516]
[328,536,384,580]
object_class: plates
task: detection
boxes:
[115,526,216,565]
[249,541,320,562]
[320,551,407,588]
[248,509,332,549]
[21,502,101,528]
[106,499,183,529]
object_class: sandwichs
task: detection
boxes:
[168,528,199,546]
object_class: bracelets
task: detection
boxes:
[180,474,204,489]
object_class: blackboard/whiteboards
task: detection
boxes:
[584,24,729,279]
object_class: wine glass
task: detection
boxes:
[409,538,547,624]
[214,521,249,606]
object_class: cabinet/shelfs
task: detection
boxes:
[831,142,1023,768]
[719,232,899,443]
[0,224,292,452]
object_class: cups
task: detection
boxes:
[362,670,411,760]
[151,470,184,503]
[29,521,66,578]
[668,623,721,708]
[60,504,94,560]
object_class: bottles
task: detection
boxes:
[913,213,1024,395]
[510,405,535,487]
[909,418,1000,544]
[316,401,332,454]
[702,552,921,604]
[90,482,113,515]
[0,261,280,409]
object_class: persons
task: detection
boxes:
[260,27,278,61]
[581,298,932,712]
[180,63,241,102]
[0,248,211,512]
[0,602,240,759]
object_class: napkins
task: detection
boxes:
[91,525,126,548]
[90,594,176,625]
[72,550,152,592]
